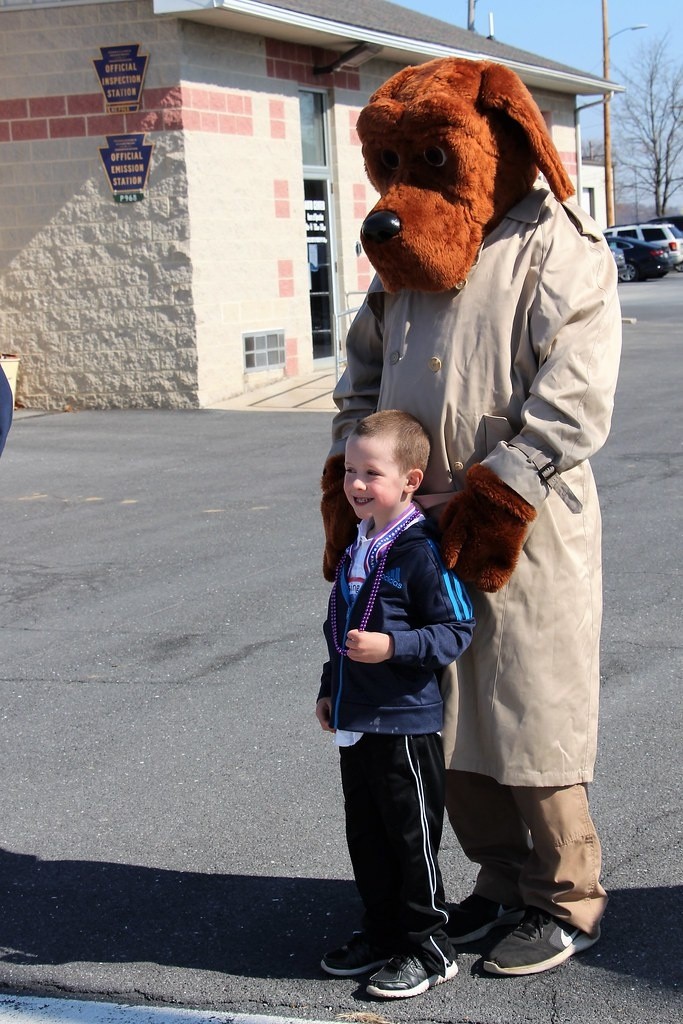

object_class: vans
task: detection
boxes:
[604,216,683,267]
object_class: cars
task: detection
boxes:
[605,235,673,282]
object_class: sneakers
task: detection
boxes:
[440,893,526,945]
[365,955,459,998]
[482,905,601,975]
[320,927,394,976]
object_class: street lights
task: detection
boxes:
[602,0,647,229]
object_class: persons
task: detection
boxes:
[319,59,622,975]
[314,409,476,1000]
